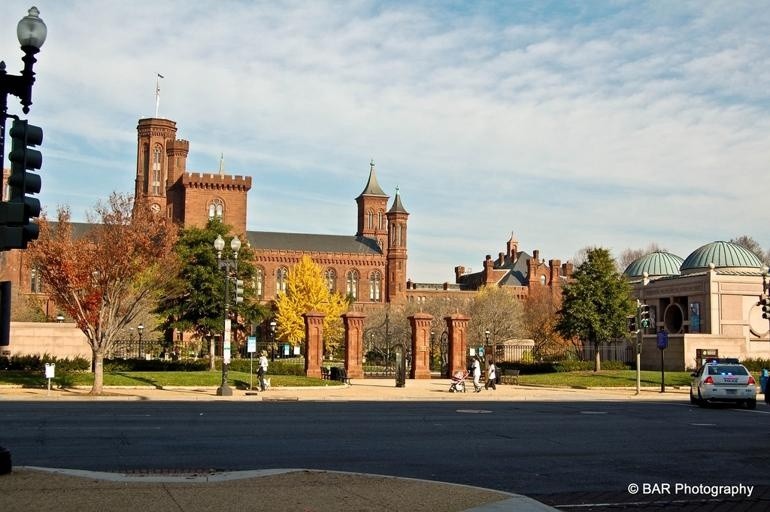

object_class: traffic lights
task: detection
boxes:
[628,316,637,331]
[10,119,43,244]
[762,298,770,319]
[640,304,650,328]
[235,278,244,303]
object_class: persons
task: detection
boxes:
[485,359,497,390]
[256,350,269,391]
[469,356,482,392]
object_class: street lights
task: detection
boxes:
[485,329,490,343]
[430,329,437,372]
[56,314,65,323]
[270,321,277,361]
[214,232,242,395]
[136,321,145,360]
[0,7,46,474]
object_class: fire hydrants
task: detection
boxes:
[759,368,768,392]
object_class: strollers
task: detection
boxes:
[449,370,471,393]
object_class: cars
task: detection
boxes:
[689,356,758,410]
